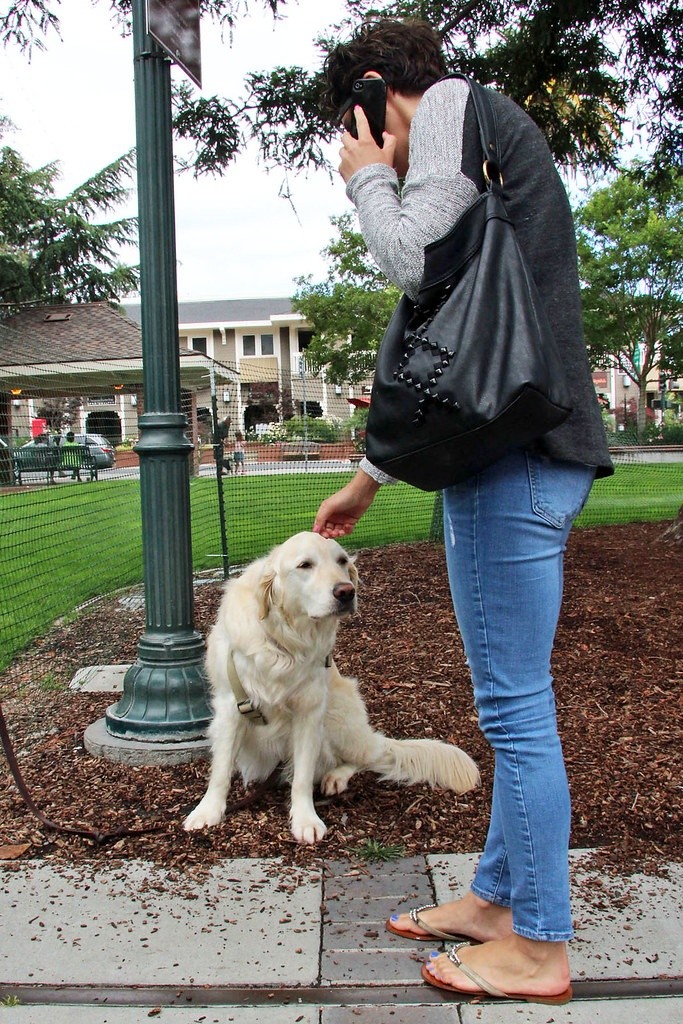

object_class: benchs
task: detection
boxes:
[0,446,99,486]
[347,452,365,468]
[283,442,320,458]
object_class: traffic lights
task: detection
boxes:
[669,376,680,391]
[658,373,666,391]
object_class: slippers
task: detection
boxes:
[384,903,484,945]
[420,941,573,1006]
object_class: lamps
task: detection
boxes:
[131,394,138,406]
[224,392,230,402]
[335,384,341,394]
[13,400,20,408]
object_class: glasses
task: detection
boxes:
[331,96,354,133]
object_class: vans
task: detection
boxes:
[14,434,115,470]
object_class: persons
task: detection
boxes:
[212,415,232,475]
[45,435,60,486]
[234,430,246,475]
[311,16,614,1005]
[61,432,83,483]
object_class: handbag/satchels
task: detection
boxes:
[365,70,576,493]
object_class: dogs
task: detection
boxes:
[178,531,483,846]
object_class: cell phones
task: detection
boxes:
[349,77,387,149]
